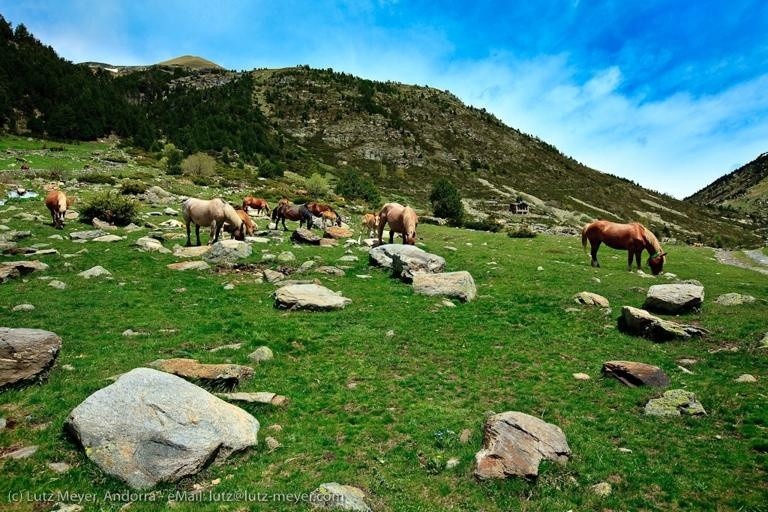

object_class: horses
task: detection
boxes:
[580,218,668,276]
[378,202,419,246]
[45,190,68,230]
[179,197,258,248]
[356,211,382,246]
[271,198,342,232]
[241,196,271,218]
